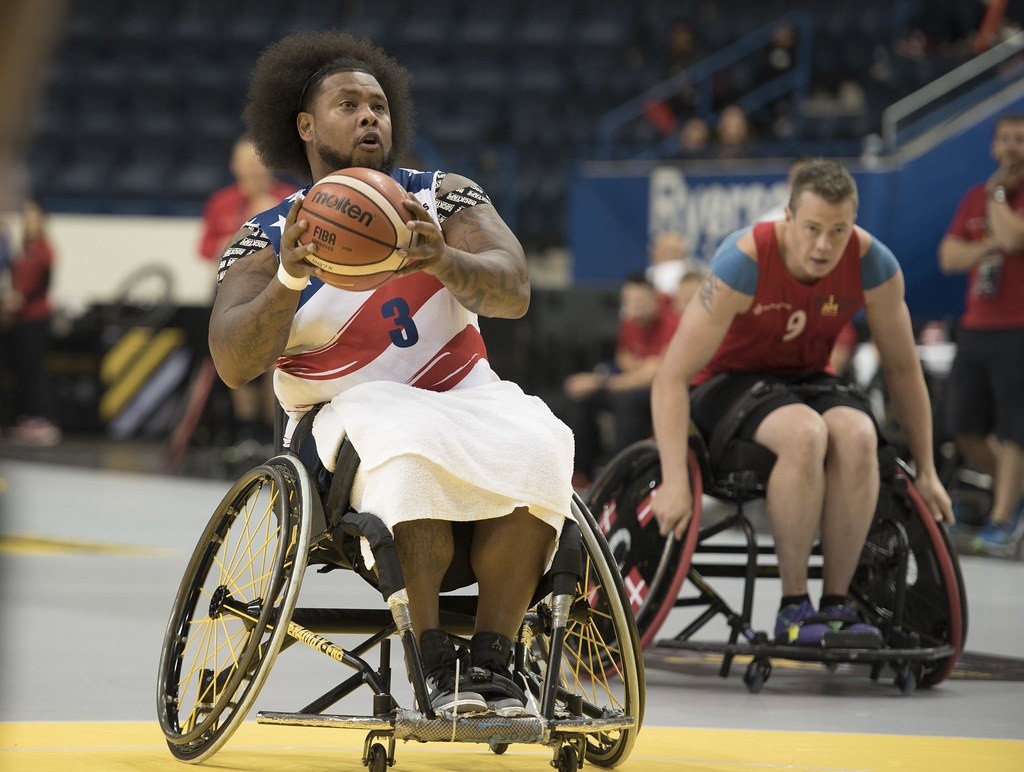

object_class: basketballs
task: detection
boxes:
[293,166,419,293]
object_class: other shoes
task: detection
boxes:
[970,524,1015,551]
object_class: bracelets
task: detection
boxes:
[598,374,609,394]
[277,262,310,290]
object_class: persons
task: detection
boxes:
[651,159,956,648]
[632,0,1006,168]
[198,130,299,462]
[938,110,1024,556]
[0,196,60,446]
[208,32,573,720]
[564,275,712,398]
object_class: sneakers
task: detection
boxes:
[775,600,830,644]
[820,604,883,644]
[470,632,526,714]
[412,628,487,713]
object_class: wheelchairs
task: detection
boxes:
[149,396,651,771]
[557,435,972,697]
